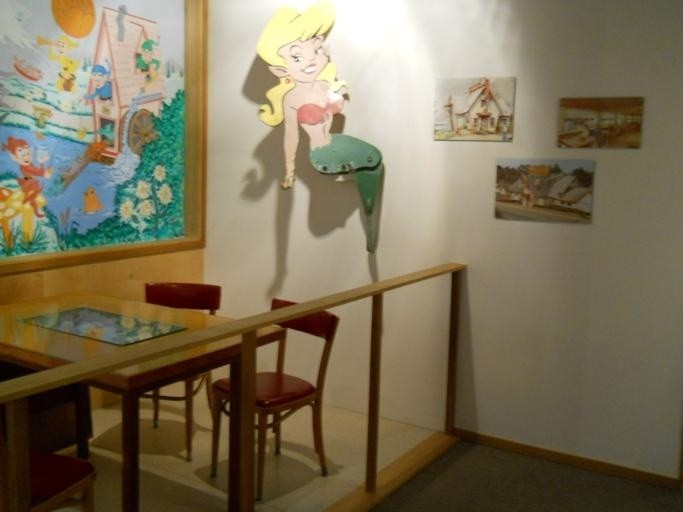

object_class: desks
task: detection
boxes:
[1,291,287,511]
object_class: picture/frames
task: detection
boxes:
[0,0,214,278]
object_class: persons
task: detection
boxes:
[256,0,384,252]
[49,36,82,94]
[136,39,160,81]
[84,65,112,100]
[7,137,45,218]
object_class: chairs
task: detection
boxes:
[211,298,340,502]
[140,283,221,462]
[3,442,98,511]
